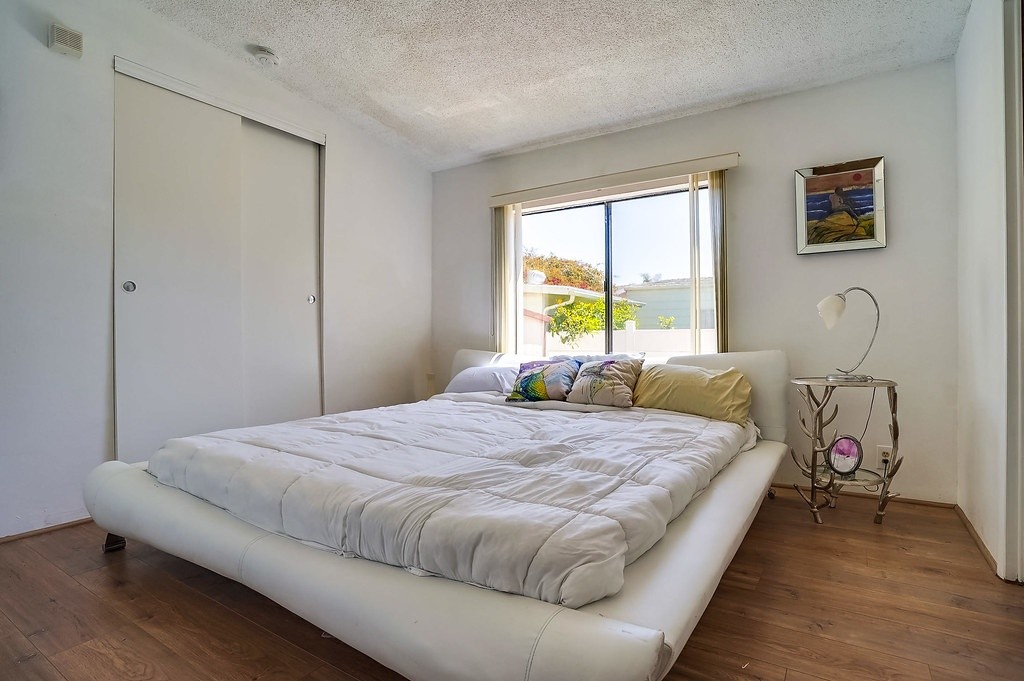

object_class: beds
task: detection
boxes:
[85,350,789,681]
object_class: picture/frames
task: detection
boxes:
[793,155,886,255]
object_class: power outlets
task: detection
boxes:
[876,445,893,470]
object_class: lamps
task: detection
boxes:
[818,286,879,384]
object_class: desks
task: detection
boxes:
[791,376,905,524]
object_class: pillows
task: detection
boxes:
[566,358,645,407]
[505,359,583,403]
[444,367,518,393]
[632,364,752,427]
